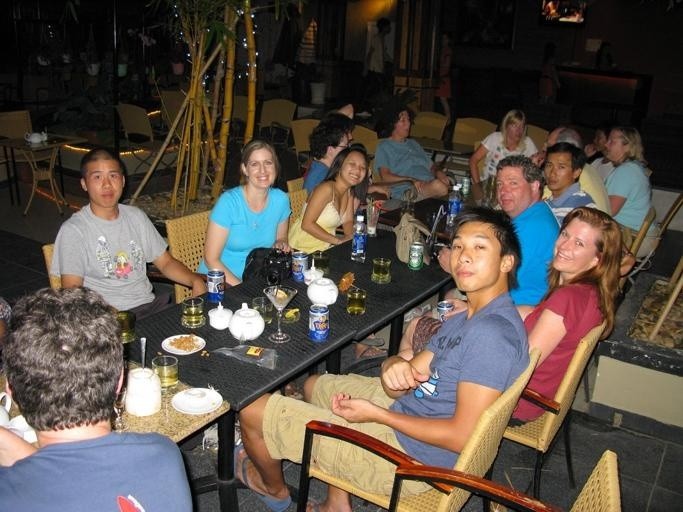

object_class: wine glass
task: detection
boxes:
[111,386,131,433]
[262,284,298,345]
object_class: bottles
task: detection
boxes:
[456,183,464,214]
[350,215,368,263]
[446,185,460,228]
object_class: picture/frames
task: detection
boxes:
[366,21,394,62]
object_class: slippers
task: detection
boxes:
[352,345,388,359]
[361,333,385,346]
[234,446,292,512]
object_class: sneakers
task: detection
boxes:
[202,424,242,449]
[404,304,431,323]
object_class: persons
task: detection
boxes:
[1,283,195,511]
[433,29,455,128]
[397,109,651,427]
[196,103,451,399]
[235,205,530,512]
[1,295,39,471]
[538,40,561,106]
[48,147,268,454]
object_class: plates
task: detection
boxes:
[171,387,224,415]
[161,334,207,356]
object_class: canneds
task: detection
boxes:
[206,269,226,305]
[407,241,425,271]
[460,175,471,196]
[291,251,309,284]
[308,303,331,344]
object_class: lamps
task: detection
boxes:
[540,1,586,24]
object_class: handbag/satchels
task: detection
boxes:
[243,248,271,282]
[393,212,432,265]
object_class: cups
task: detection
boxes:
[346,288,367,317]
[311,251,331,277]
[303,258,325,286]
[251,297,273,325]
[116,310,137,345]
[207,302,233,331]
[181,296,205,328]
[40,131,48,142]
[371,257,392,285]
[124,367,162,417]
[399,200,416,219]
[366,203,381,238]
[152,355,180,391]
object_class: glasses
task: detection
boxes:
[336,145,349,149]
[248,160,273,168]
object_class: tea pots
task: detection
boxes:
[0,391,12,427]
[228,302,265,341]
[23,132,44,144]
[306,276,339,307]
